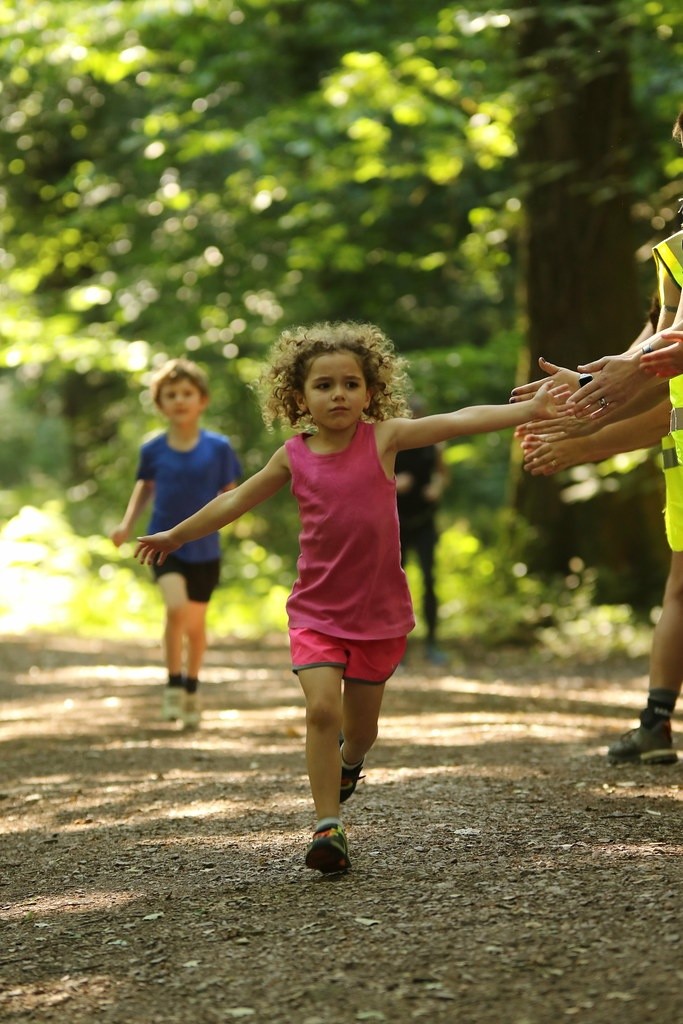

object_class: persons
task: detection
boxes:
[394,405,448,645]
[509,109,683,763]
[111,359,242,729]
[133,324,575,874]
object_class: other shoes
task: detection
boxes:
[162,684,182,722]
[183,692,199,728]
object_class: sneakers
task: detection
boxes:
[608,713,678,764]
[336,738,362,803]
[305,820,351,875]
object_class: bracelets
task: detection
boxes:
[643,345,652,354]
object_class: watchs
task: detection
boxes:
[579,374,593,387]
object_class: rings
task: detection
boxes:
[600,398,607,406]
[551,460,558,467]
